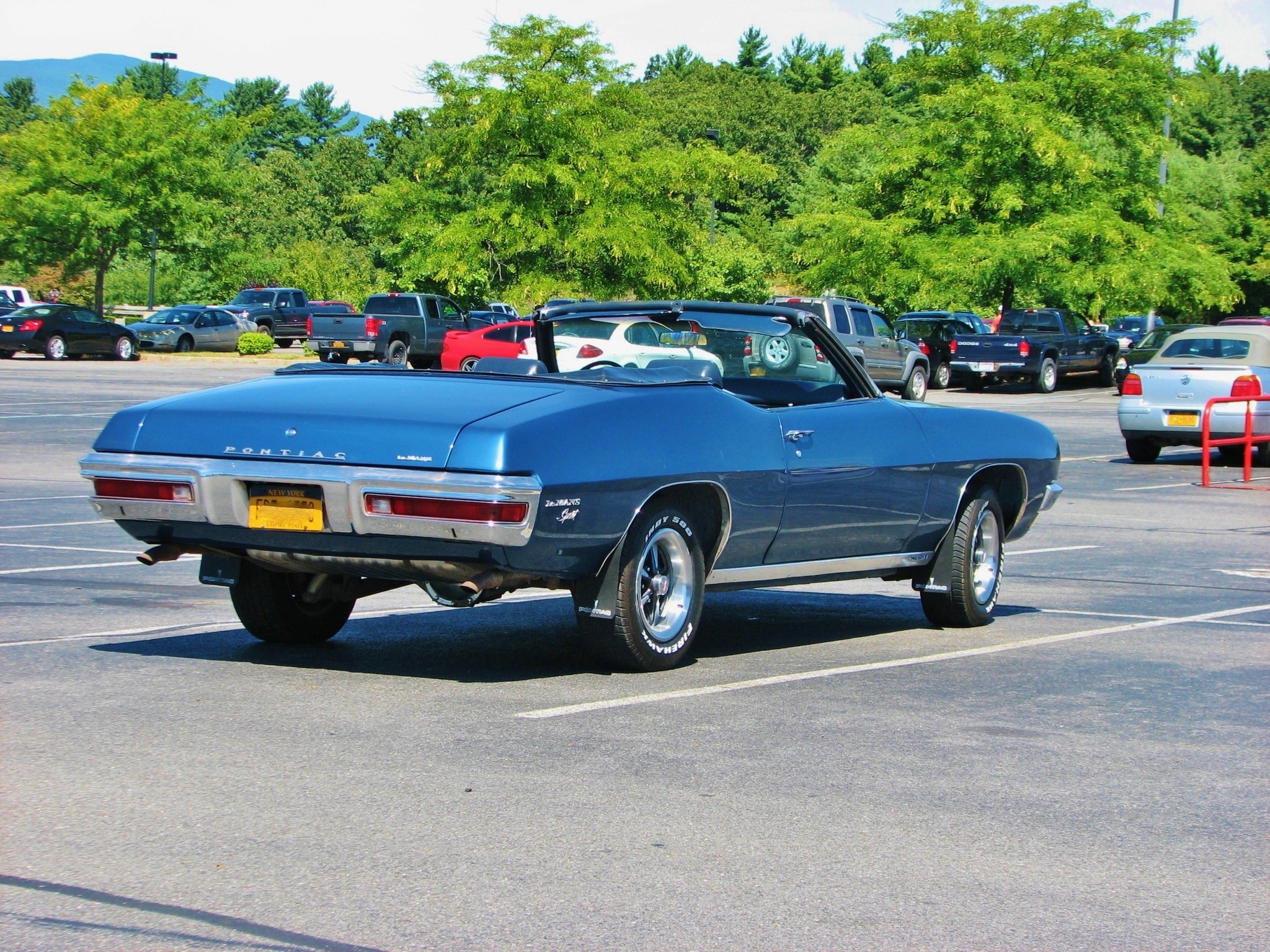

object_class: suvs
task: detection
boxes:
[1079,316,1169,360]
[520,299,596,320]
[742,297,932,404]
[649,313,747,377]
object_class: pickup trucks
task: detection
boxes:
[211,287,351,349]
[0,285,50,315]
[303,292,494,369]
[949,307,1120,394]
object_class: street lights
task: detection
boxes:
[148,52,176,314]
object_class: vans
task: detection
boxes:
[487,304,520,323]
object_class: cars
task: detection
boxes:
[308,301,357,313]
[518,316,726,375]
[1219,316,1270,326]
[440,320,534,372]
[79,298,1064,669]
[0,291,39,315]
[125,303,259,353]
[1117,325,1270,462]
[0,304,141,361]
[446,312,517,324]
[891,311,991,388]
[1114,322,1247,395]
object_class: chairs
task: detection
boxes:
[647,358,722,390]
[473,355,547,375]
